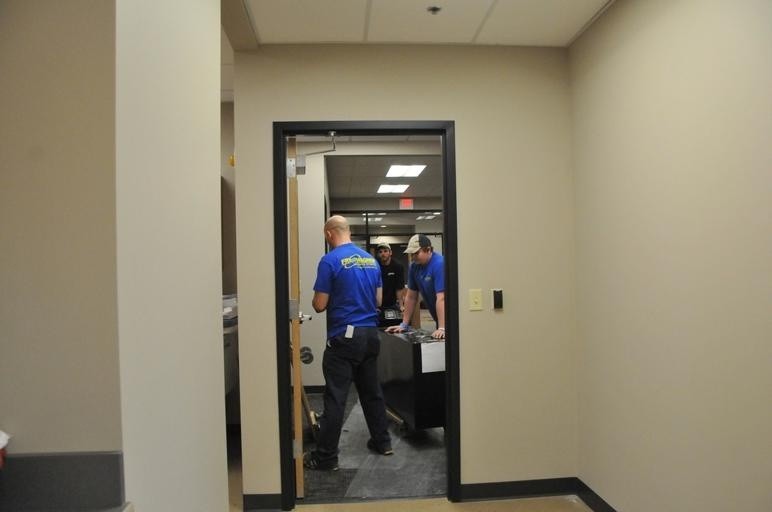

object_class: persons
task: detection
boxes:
[383,233,447,340]
[303,215,393,472]
[374,241,406,326]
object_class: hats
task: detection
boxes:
[377,242,391,250]
[402,234,431,257]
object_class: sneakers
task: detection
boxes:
[367,438,394,455]
[303,452,339,471]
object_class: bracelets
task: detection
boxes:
[437,328,445,331]
[400,322,408,331]
